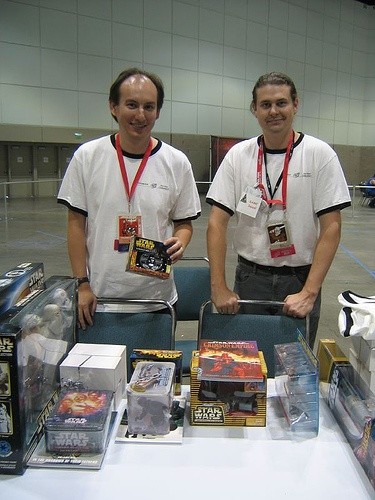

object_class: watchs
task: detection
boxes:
[75,277,91,290]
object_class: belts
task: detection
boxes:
[239,255,311,275]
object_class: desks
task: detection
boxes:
[0,378,374,500]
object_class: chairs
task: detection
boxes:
[197,300,309,380]
[171,256,213,377]
[73,297,175,383]
[360,182,375,207]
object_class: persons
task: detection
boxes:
[58,68,202,382]
[366,173,375,208]
[205,71,352,351]
[14,289,72,423]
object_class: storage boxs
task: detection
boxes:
[0,262,78,475]
[42,328,268,453]
[316,338,375,492]
